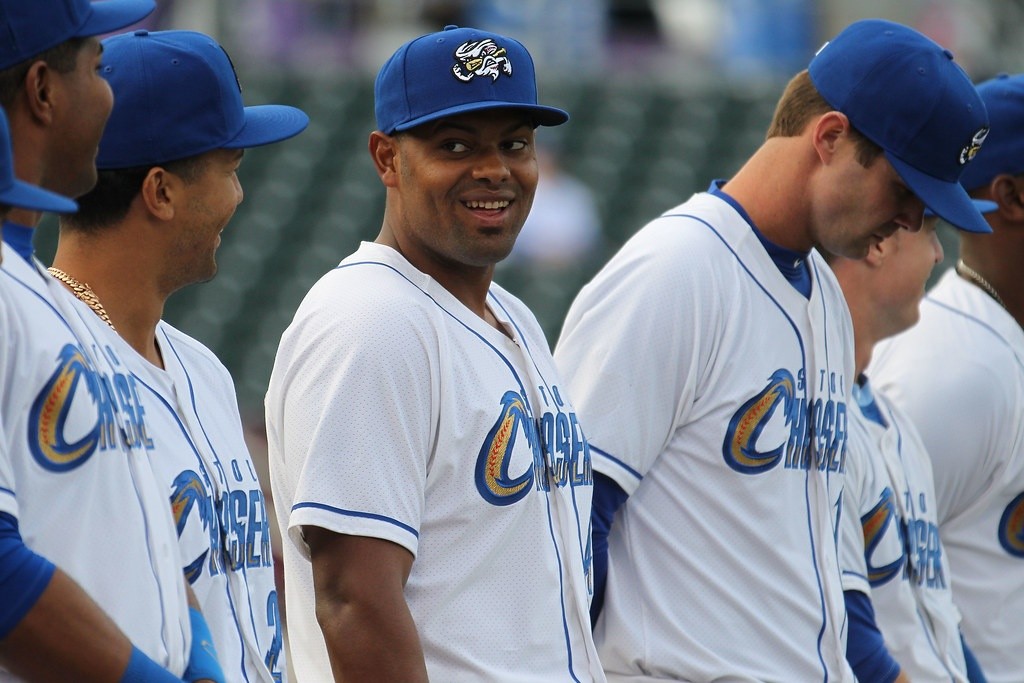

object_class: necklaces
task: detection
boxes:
[954,256,1011,313]
[41,266,119,341]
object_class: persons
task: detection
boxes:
[265,24,605,683]
[550,19,1024,683]
[0,1,311,683]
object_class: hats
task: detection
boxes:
[373,23,569,132]
[0,107,80,213]
[0,0,157,69]
[920,195,998,219]
[807,19,992,234]
[960,72,1024,186]
[95,28,308,168]
